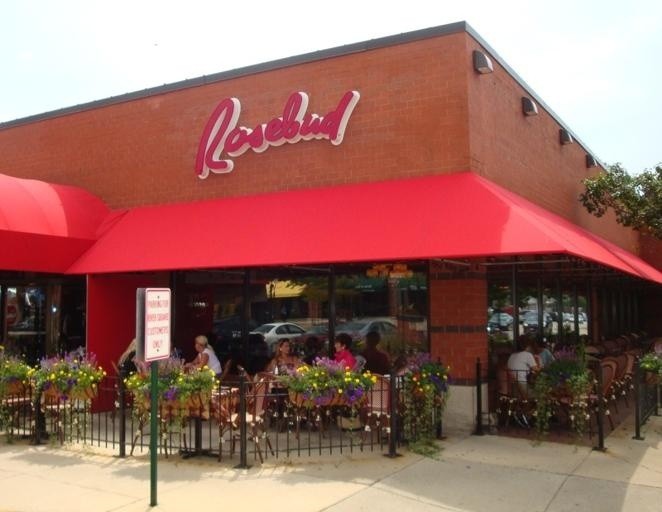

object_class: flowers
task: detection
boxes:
[529,342,591,452]
[0,343,106,402]
[123,353,216,434]
[400,352,452,463]
[637,351,662,382]
[279,356,378,438]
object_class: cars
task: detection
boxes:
[335,317,396,342]
[250,321,326,359]
[488,308,588,336]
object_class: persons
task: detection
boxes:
[302,337,323,367]
[360,332,389,374]
[192,335,223,377]
[267,339,300,372]
[117,338,137,378]
[530,336,556,368]
[333,334,357,368]
[507,336,543,428]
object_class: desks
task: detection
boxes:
[159,385,240,460]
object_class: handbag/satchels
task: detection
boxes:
[336,414,362,432]
[120,351,137,379]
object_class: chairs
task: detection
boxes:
[217,359,406,465]
[496,330,649,430]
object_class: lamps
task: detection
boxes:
[520,96,537,117]
[559,128,573,145]
[585,152,597,169]
[474,48,494,76]
